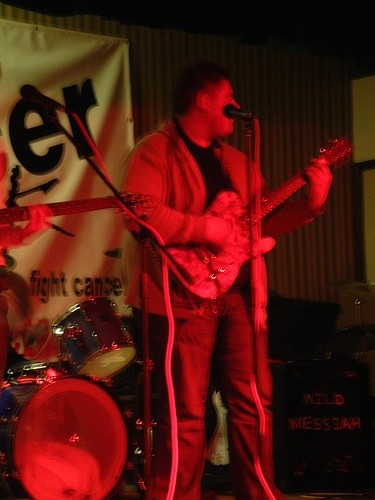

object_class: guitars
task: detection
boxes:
[0,189,144,226]
[147,136,355,304]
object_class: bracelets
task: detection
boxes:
[301,190,326,220]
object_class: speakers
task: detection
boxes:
[285,359,371,487]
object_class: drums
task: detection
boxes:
[51,296,134,380]
[1,362,131,500]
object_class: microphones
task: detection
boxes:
[223,104,253,119]
[19,85,72,116]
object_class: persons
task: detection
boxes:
[117,61,333,500]
